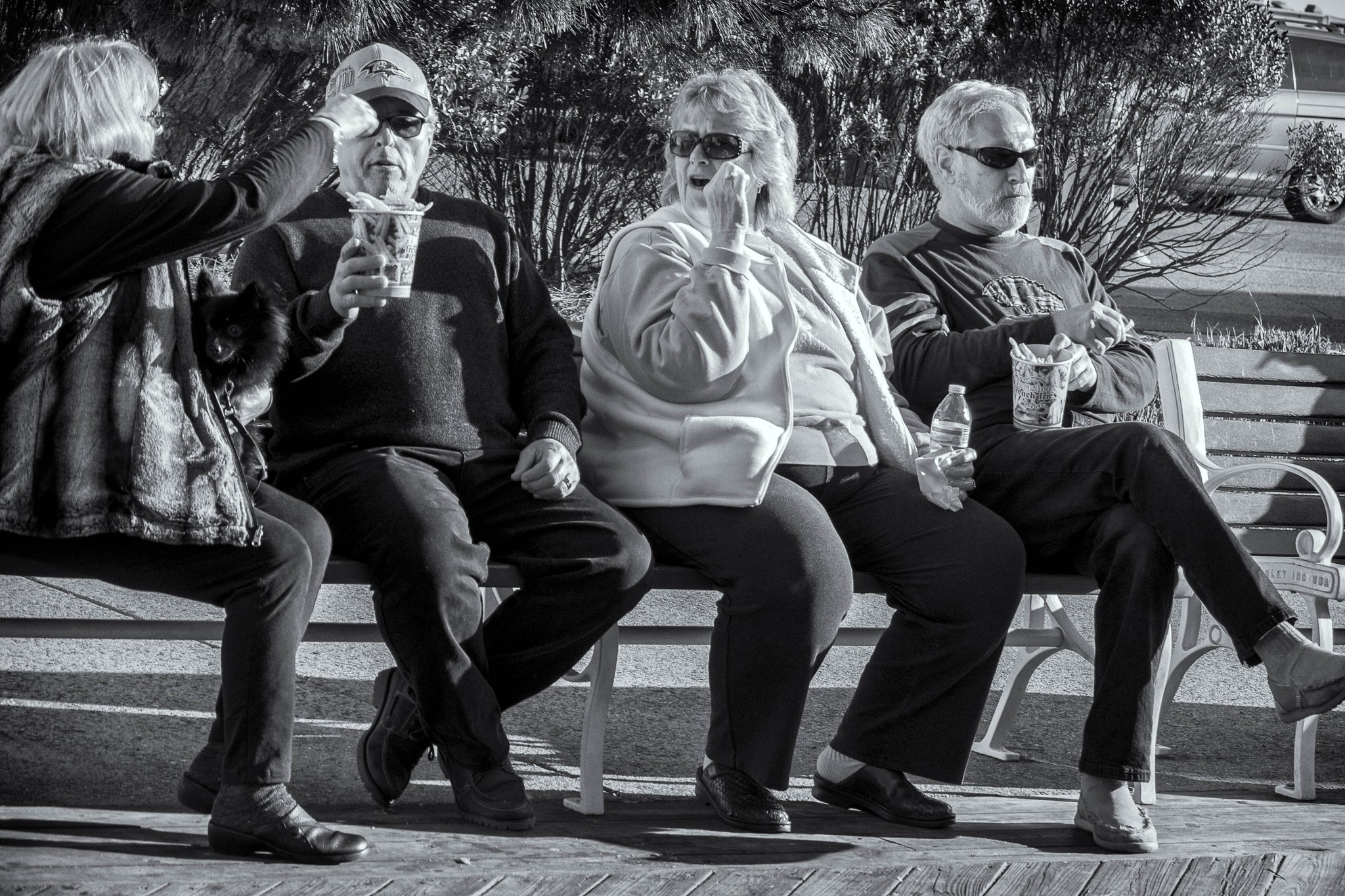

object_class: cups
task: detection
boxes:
[348,209,425,298]
[1011,344,1074,430]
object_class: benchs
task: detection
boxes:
[0,337,1345,817]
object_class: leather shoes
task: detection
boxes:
[211,807,365,862]
[812,756,955,829]
[180,757,227,812]
[688,760,795,836]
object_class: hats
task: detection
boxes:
[325,42,432,118]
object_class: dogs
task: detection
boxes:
[189,268,290,438]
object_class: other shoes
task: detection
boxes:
[1073,789,1160,852]
[1267,641,1345,725]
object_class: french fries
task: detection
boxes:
[351,213,421,278]
[1125,318,1135,332]
[1008,338,1054,364]
[1013,362,1070,425]
[345,188,434,211]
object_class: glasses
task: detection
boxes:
[369,116,424,138]
[952,144,1044,170]
[669,131,754,159]
[147,106,164,129]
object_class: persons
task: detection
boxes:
[575,67,1024,838]
[855,78,1344,852]
[224,41,651,830]
[0,39,378,862]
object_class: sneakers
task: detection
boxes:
[354,667,426,801]
[439,738,538,835]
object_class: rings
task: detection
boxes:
[564,477,571,488]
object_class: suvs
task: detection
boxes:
[1111,5,1344,226]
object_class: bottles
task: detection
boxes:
[928,384,972,468]
